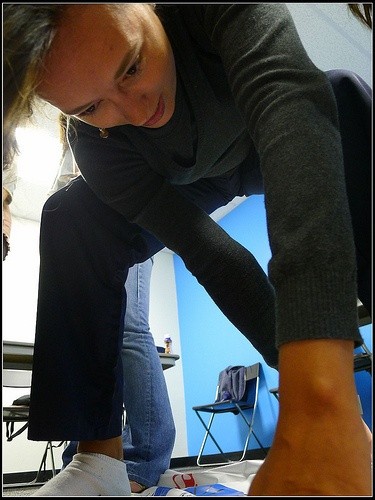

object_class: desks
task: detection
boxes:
[3,339,180,372]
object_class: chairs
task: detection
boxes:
[192,362,269,467]
[3,405,67,484]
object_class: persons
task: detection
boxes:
[3,1,375,496]
[49,113,176,493]
[4,120,20,262]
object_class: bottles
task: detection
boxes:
[164,337,173,354]
